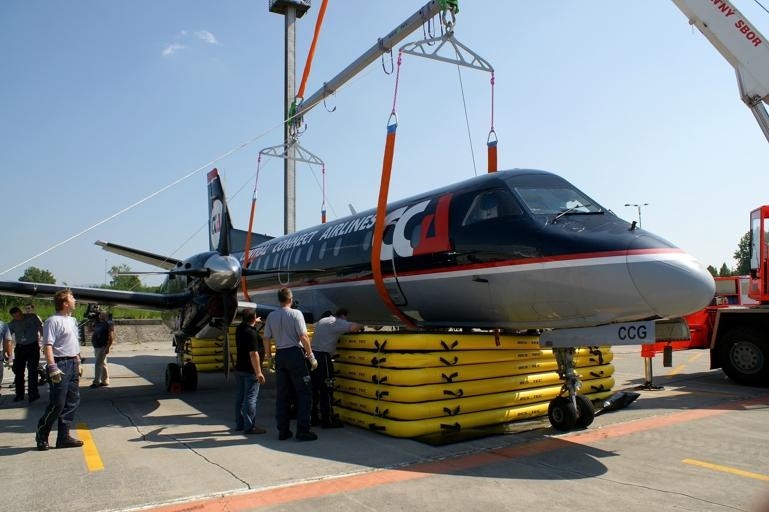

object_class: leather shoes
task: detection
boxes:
[100,382,109,386]
[89,384,100,389]
[245,428,267,435]
[28,393,40,402]
[13,396,25,402]
[295,431,318,442]
[279,430,293,441]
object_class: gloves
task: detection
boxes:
[78,359,83,377]
[305,352,318,371]
[47,363,64,384]
[7,355,14,367]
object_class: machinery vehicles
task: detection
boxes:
[288,0,769,393]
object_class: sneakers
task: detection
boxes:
[326,422,342,429]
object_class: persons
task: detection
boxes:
[7,309,44,401]
[313,310,364,425]
[236,309,265,436]
[88,311,115,387]
[266,287,317,442]
[0,319,14,396]
[24,305,43,339]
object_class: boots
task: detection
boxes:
[56,424,83,448]
[35,431,49,450]
